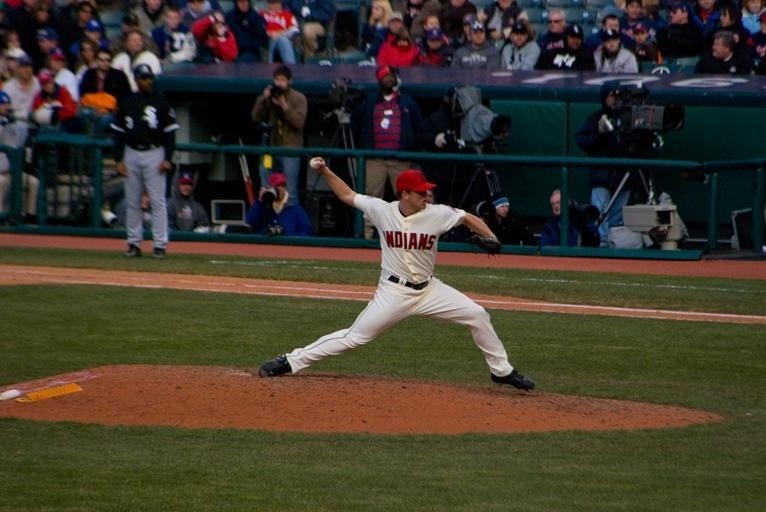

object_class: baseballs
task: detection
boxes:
[309,158,321,168]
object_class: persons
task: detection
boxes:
[1,0,766,247]
[108,61,180,262]
[257,154,537,392]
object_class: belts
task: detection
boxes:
[388,275,435,289]
[128,145,161,151]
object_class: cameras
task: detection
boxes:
[271,85,281,98]
[261,188,277,203]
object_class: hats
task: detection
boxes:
[0,12,154,104]
[396,169,436,191]
[386,12,650,46]
[375,65,391,82]
[177,172,193,183]
[491,192,510,209]
[756,12,766,24]
[268,173,287,185]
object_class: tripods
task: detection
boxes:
[304,112,359,229]
[453,167,500,208]
[594,168,650,232]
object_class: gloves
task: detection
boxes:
[480,234,502,253]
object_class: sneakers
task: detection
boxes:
[491,368,535,391]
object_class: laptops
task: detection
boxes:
[210,200,255,226]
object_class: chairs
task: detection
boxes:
[472,1,710,76]
